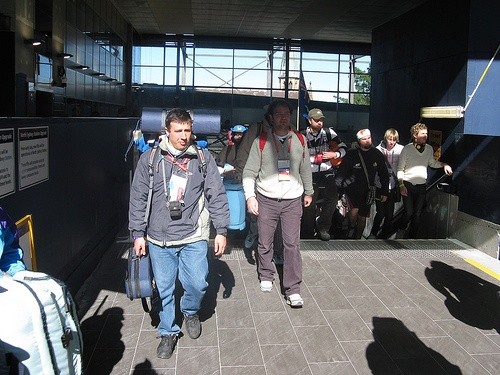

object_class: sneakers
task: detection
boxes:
[184,313,201,339]
[156,334,177,359]
[286,293,303,307]
[319,229,330,240]
[260,280,272,292]
[245,234,255,248]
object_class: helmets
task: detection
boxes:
[231,125,246,142]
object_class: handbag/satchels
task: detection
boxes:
[367,185,376,205]
[392,178,401,203]
[125,244,153,301]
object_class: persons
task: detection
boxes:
[215,108,454,241]
[0,207,27,279]
[242,100,314,306]
[129,109,230,358]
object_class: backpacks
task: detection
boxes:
[0,270,84,375]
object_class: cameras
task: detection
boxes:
[168,201,182,220]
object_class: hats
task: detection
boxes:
[308,108,325,120]
[356,128,370,140]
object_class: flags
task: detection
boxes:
[300,72,311,121]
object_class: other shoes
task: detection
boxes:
[348,228,354,238]
